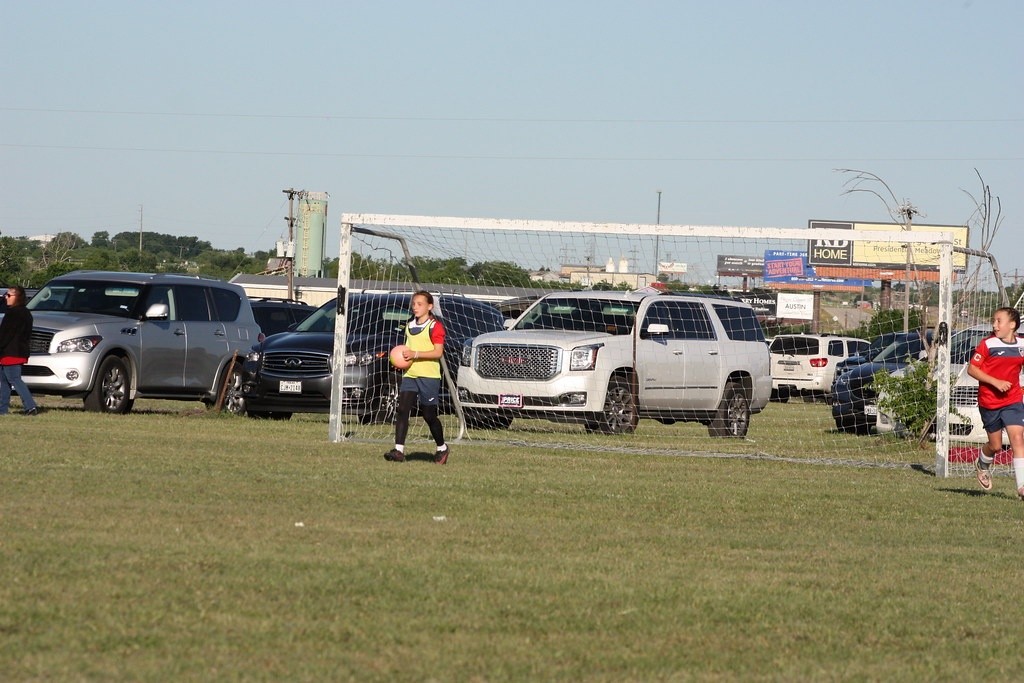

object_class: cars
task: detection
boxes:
[877,323,1024,448]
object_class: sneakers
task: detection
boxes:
[431,443,450,465]
[1014,482,1024,501]
[974,455,993,491]
[384,448,406,463]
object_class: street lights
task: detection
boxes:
[653,187,662,276]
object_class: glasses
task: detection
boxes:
[4,293,15,299]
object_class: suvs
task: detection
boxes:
[453,292,776,444]
[241,293,519,431]
[767,330,873,405]
[10,268,265,418]
[831,333,913,384]
[833,331,960,435]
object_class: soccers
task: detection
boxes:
[390,345,413,369]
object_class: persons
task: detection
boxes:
[384,291,451,465]
[0,286,38,416]
[967,307,1024,500]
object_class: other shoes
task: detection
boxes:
[19,407,39,416]
